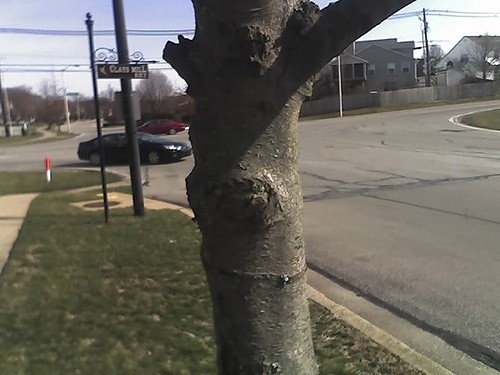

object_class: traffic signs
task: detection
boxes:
[97,64,149,79]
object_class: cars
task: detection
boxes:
[138,119,185,135]
[78,133,191,166]
